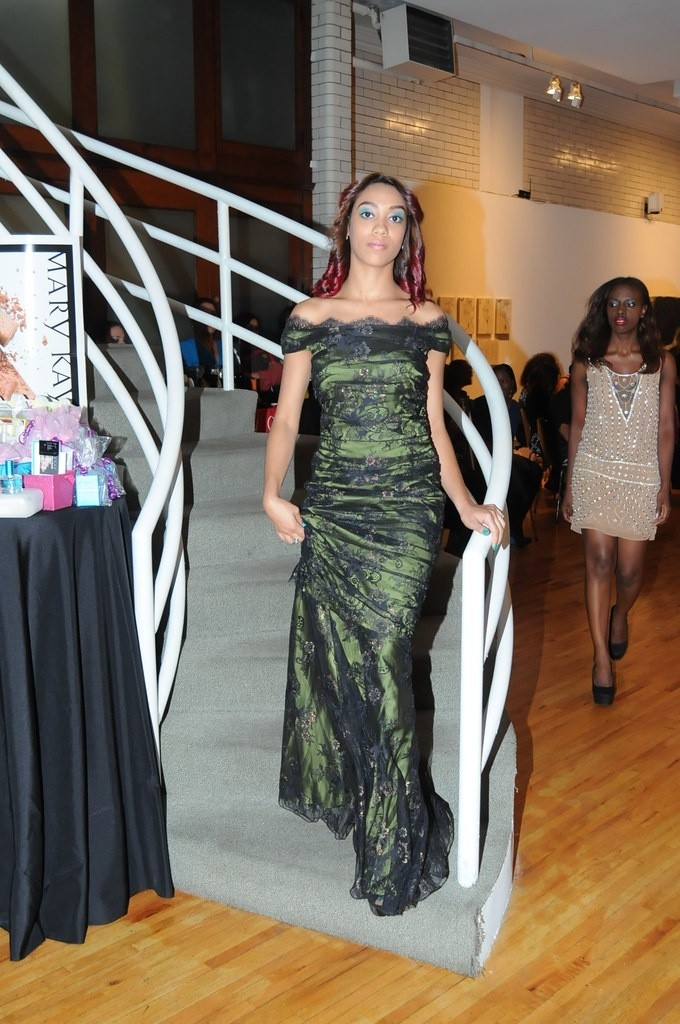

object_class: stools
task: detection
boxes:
[534,458,567,520]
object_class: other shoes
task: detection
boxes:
[510,526,532,548]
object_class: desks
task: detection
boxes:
[0,494,174,962]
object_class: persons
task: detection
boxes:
[261,175,504,915]
[560,274,675,707]
[181,297,292,393]
[93,322,126,345]
[439,348,572,554]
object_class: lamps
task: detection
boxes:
[546,75,562,102]
[567,81,582,108]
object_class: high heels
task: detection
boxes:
[609,605,629,661]
[592,662,615,706]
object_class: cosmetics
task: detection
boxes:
[0,438,62,495]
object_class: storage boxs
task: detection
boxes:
[0,487,45,519]
[76,466,112,506]
[22,471,75,511]
